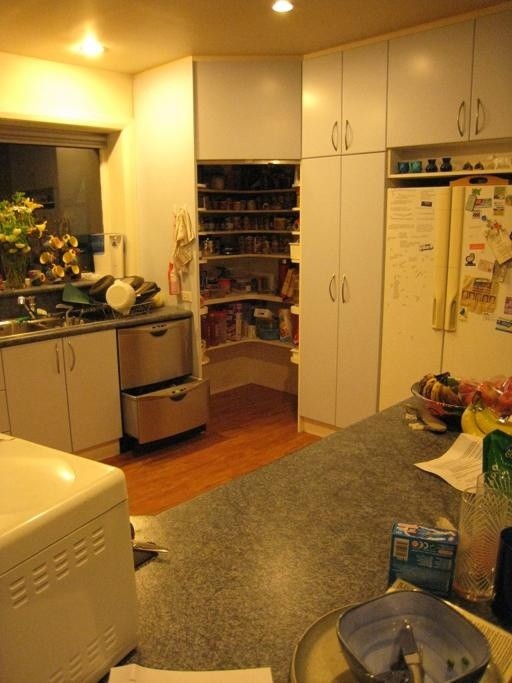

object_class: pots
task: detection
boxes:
[90,274,160,317]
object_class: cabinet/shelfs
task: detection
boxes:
[1,330,123,462]
[302,11,512,179]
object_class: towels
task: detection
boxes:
[169,208,195,273]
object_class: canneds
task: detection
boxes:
[198,193,297,256]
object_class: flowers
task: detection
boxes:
[0,191,47,289]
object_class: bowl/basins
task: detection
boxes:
[336,589,492,683]
[411,380,480,422]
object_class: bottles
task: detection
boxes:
[440,158,452,172]
[426,159,438,172]
[201,301,250,349]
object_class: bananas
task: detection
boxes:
[462,391,512,435]
[419,372,461,404]
[404,402,448,433]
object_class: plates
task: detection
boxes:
[290,602,499,683]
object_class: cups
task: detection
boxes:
[398,162,409,173]
[469,470,512,573]
[411,161,422,173]
[247,325,257,340]
[451,484,509,604]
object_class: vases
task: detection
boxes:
[1,249,30,288]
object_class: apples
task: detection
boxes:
[459,376,511,411]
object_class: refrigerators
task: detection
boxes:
[375,183,512,413]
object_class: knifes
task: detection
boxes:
[400,622,425,683]
